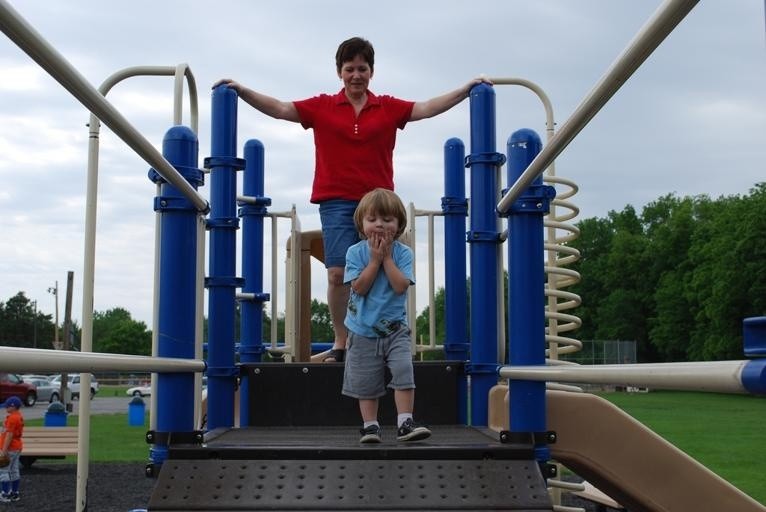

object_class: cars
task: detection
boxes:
[0,370,99,409]
[124,381,152,398]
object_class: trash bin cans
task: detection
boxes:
[127,397,146,426]
[44,402,68,426]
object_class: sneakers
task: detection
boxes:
[396,417,431,442]
[1,490,19,503]
[358,424,382,442]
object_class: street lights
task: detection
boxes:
[31,298,38,348]
[47,280,61,345]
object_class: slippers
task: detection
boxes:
[323,347,345,364]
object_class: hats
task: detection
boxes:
[1,396,22,408]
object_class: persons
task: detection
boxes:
[340,187,434,443]
[0,395,24,503]
[209,36,501,362]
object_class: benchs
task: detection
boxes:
[19,425,78,468]
